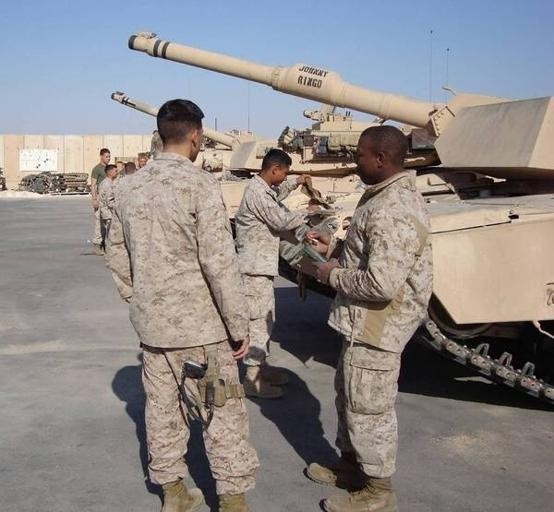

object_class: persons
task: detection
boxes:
[309,126,435,511]
[234,150,322,399]
[106,100,258,509]
[100,164,118,254]
[123,162,135,177]
[140,155,151,170]
[90,148,112,249]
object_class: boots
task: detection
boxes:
[217,492,253,512]
[160,478,204,512]
[305,450,358,488]
[260,363,289,385]
[242,366,284,399]
[321,473,398,512]
[93,244,105,256]
